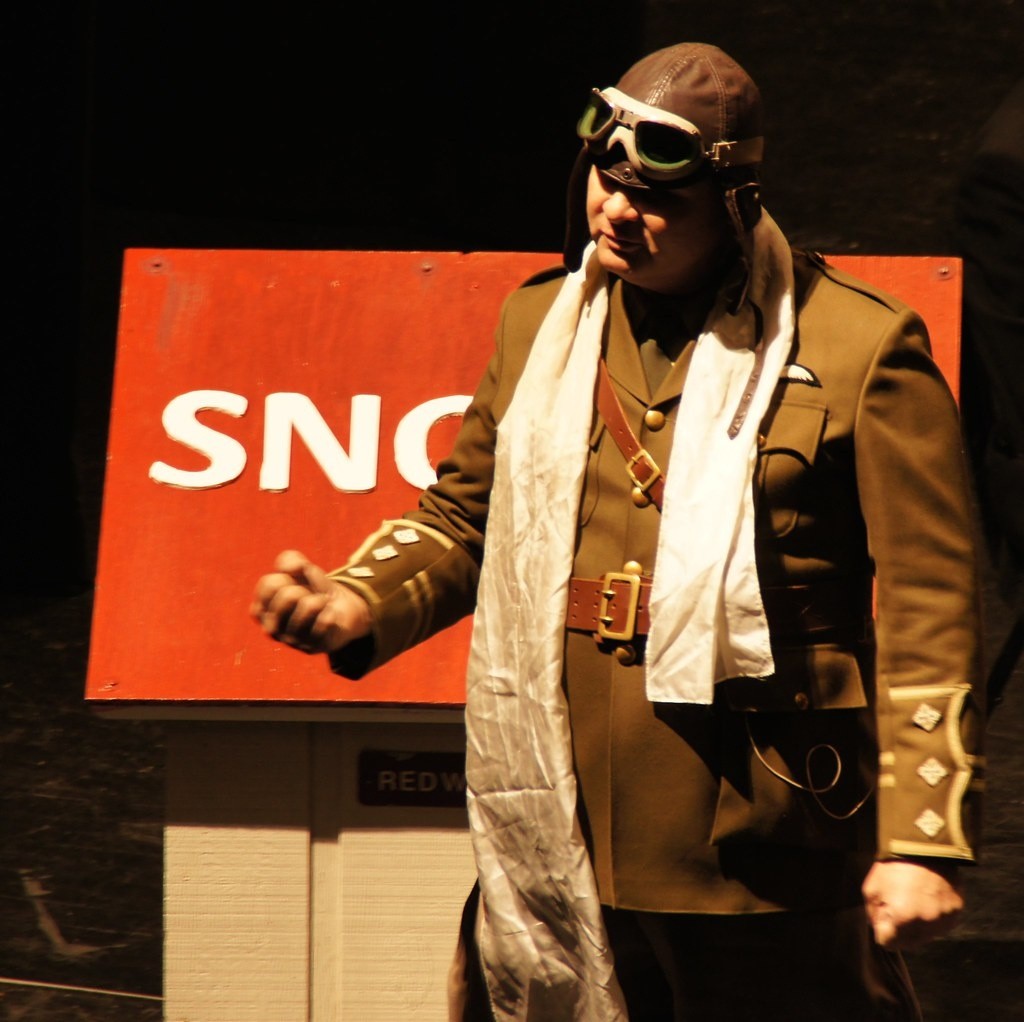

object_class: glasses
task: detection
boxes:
[578,85,711,180]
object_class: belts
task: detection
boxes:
[566,574,873,641]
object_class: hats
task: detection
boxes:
[563,42,767,313]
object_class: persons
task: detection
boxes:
[250,42,986,1022]
